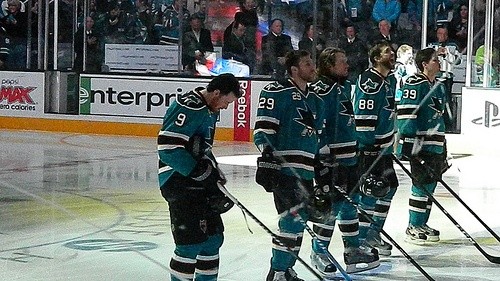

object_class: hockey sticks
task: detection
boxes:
[335,185,435,281]
[414,155,500,242]
[292,211,353,281]
[216,181,324,281]
[391,153,500,264]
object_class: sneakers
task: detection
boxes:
[310,250,337,278]
[404,223,427,245]
[421,224,440,242]
[359,243,379,256]
[343,251,380,274]
[366,231,393,256]
[265,266,306,281]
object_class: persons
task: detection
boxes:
[476,34,499,86]
[394,44,417,89]
[235,0,259,25]
[182,17,215,69]
[447,4,468,46]
[396,47,448,243]
[427,27,462,134]
[261,18,296,75]
[156,73,244,281]
[351,42,397,254]
[251,50,326,281]
[0,0,206,59]
[338,24,368,57]
[298,21,326,57]
[311,46,380,276]
[370,20,401,46]
[370,0,439,32]
[222,20,255,63]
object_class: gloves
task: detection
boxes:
[357,146,385,176]
[192,161,225,195]
[399,135,420,161]
[255,155,283,193]
[314,157,339,188]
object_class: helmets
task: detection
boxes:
[308,185,332,222]
[357,173,390,199]
[204,191,233,214]
